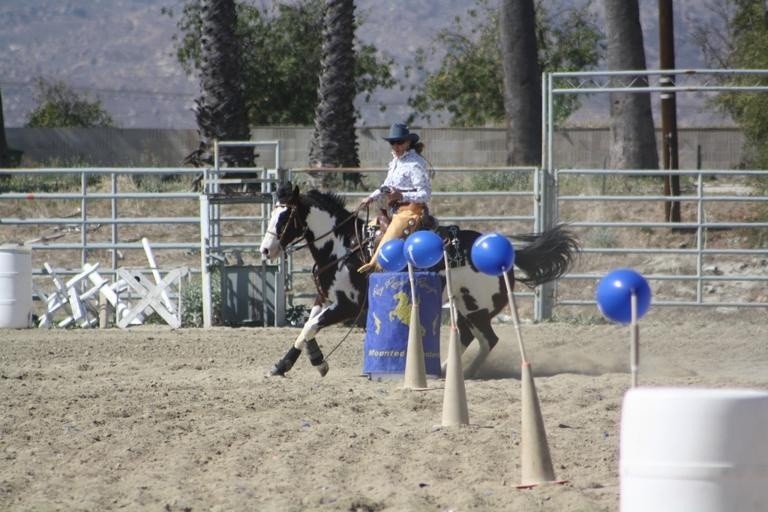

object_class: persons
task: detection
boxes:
[358,121,437,274]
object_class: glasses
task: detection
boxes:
[389,140,406,145]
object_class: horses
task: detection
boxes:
[259,181,584,380]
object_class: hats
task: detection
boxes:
[383,122,419,144]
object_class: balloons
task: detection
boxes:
[375,238,411,274]
[402,230,445,270]
[470,232,516,277]
[595,268,651,325]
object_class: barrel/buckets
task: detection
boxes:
[618,386,768,512]
[0,248,33,329]
